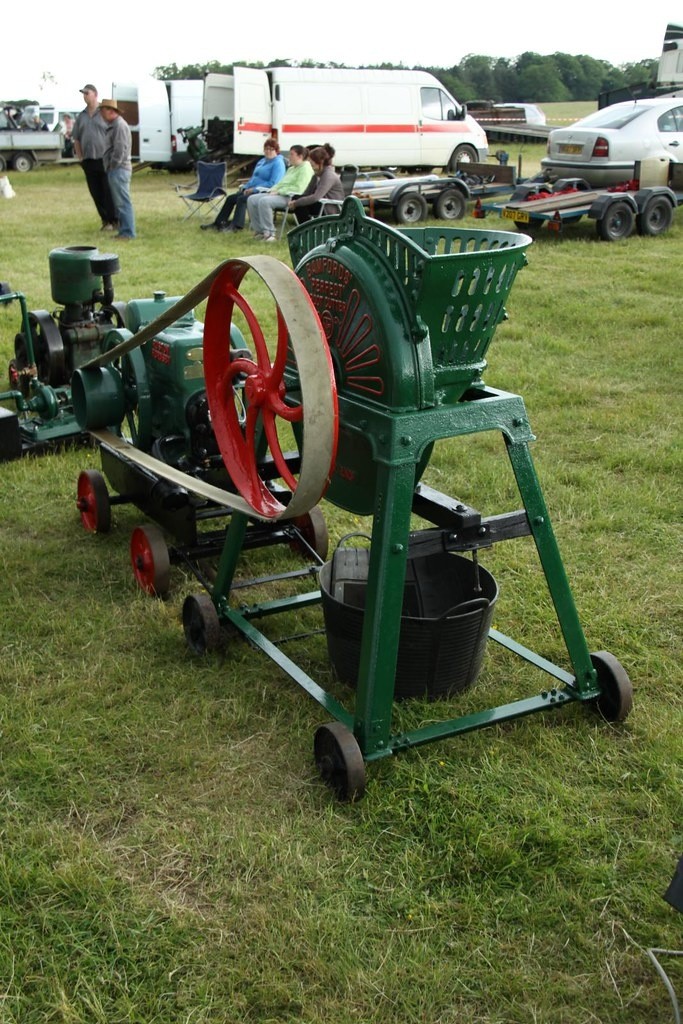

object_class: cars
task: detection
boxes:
[540,97,683,189]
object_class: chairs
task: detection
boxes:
[169,161,229,225]
[280,164,359,242]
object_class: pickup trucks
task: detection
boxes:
[0,126,64,174]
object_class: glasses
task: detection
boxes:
[264,148,275,152]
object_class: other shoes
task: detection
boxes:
[110,229,134,242]
[99,223,118,231]
[201,220,224,230]
[217,223,238,233]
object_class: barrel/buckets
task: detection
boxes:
[320,533,499,699]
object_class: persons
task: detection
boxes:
[200,137,287,235]
[246,142,346,244]
[100,99,137,239]
[70,83,119,230]
[33,113,77,159]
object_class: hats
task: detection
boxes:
[97,99,124,113]
[79,84,98,93]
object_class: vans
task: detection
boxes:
[203,66,489,176]
[116,76,203,164]
[19,103,84,134]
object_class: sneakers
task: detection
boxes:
[253,230,276,242]
